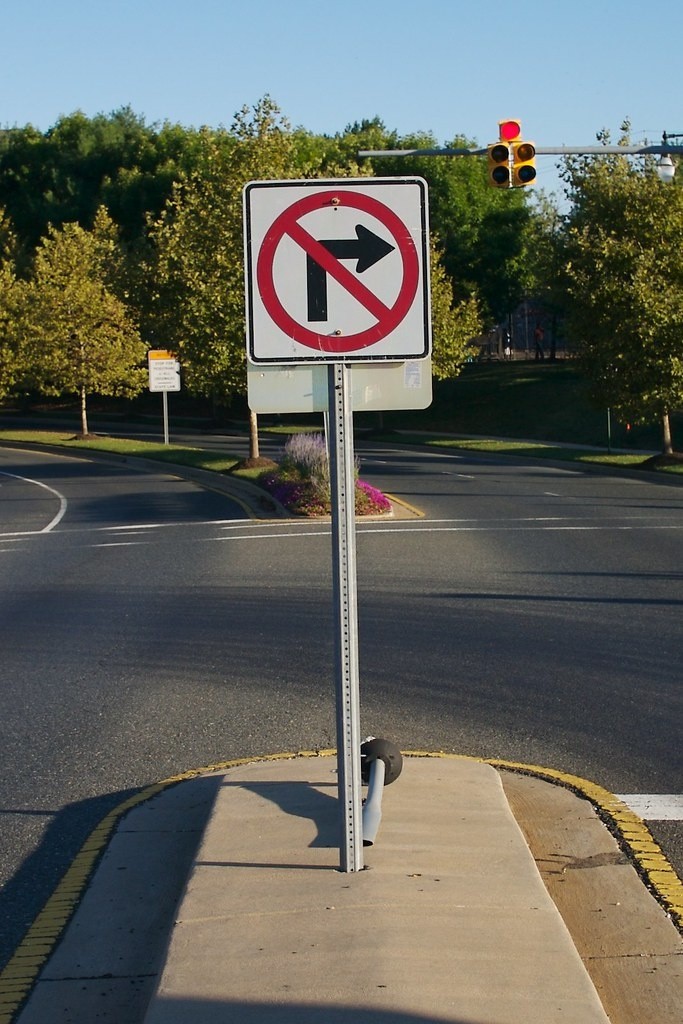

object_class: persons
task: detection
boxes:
[534,320,545,360]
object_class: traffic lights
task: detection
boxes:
[498,120,521,142]
[489,143,512,188]
[513,142,537,187]
